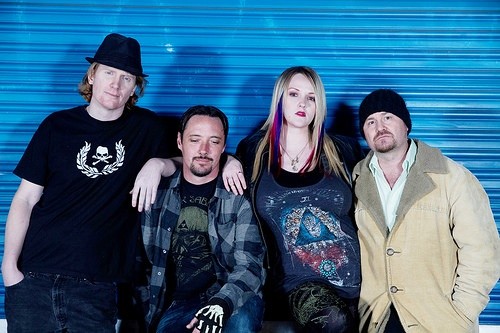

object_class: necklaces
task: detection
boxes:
[279,139,310,171]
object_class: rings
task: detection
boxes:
[237,171,243,174]
[226,177,233,179]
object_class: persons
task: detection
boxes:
[0,32,183,333]
[132,105,265,333]
[222,66,362,333]
[351,89,500,333]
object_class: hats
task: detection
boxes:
[359,89,411,140]
[85,33,149,77]
